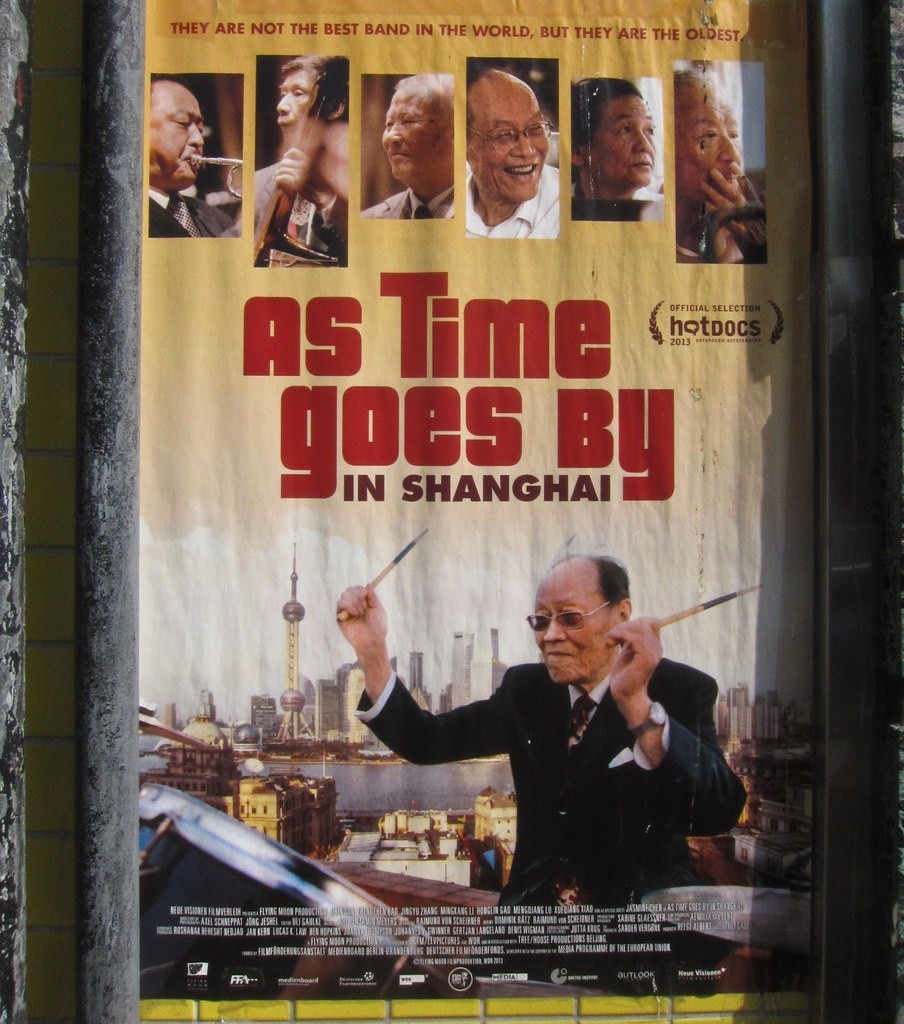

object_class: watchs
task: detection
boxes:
[628,701,666,737]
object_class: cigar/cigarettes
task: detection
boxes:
[727,175,745,179]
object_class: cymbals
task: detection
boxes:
[138,711,210,752]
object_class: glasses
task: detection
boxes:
[462,115,559,151]
[526,598,614,631]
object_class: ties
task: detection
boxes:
[169,195,203,239]
[568,694,598,748]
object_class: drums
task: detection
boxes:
[640,885,810,992]
[136,781,619,999]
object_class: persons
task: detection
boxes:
[255,54,351,267]
[571,77,664,200]
[361,74,454,219]
[338,556,745,994]
[674,69,767,264]
[149,74,242,238]
[466,57,560,240]
